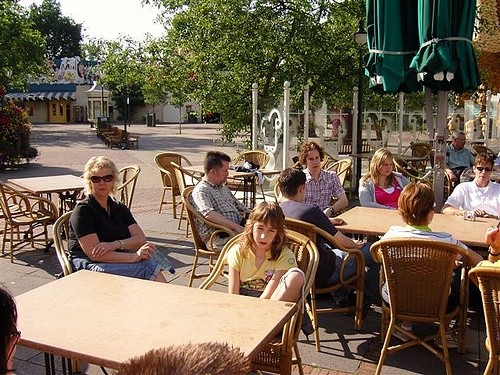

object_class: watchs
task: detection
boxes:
[119,240,125,250]
[487,246,500,256]
[330,206,336,215]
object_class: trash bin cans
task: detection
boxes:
[187,110,198,122]
[98,116,109,128]
[146,112,155,126]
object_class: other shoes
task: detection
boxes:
[392,321,415,342]
[434,328,458,348]
[334,292,358,307]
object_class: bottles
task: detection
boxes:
[149,250,175,274]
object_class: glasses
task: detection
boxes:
[12,330,21,344]
[477,167,492,172]
[91,174,114,183]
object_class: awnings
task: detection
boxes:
[5,83,77,103]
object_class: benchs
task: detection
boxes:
[335,139,382,157]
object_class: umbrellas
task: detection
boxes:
[365,0,424,156]
[408,0,481,213]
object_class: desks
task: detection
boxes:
[8,173,84,250]
[348,152,400,172]
[333,206,500,259]
[13,269,299,375]
[183,165,283,209]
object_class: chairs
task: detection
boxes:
[467,266,500,375]
[443,171,453,197]
[393,156,427,182]
[115,163,142,210]
[326,157,353,188]
[95,126,140,151]
[0,181,59,262]
[169,162,203,238]
[471,146,499,160]
[181,185,245,288]
[408,140,435,170]
[284,217,366,353]
[197,227,319,375]
[57,175,86,233]
[51,210,73,278]
[232,150,271,202]
[368,238,472,375]
[0,183,29,240]
[153,153,205,218]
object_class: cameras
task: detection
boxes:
[464,210,476,221]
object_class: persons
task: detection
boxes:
[68,156,172,284]
[277,168,374,316]
[440,153,500,218]
[114,341,261,375]
[445,130,475,186]
[485,227,500,263]
[358,147,410,210]
[223,202,306,301]
[298,140,348,217]
[188,151,252,251]
[380,182,483,350]
[0,287,21,375]
